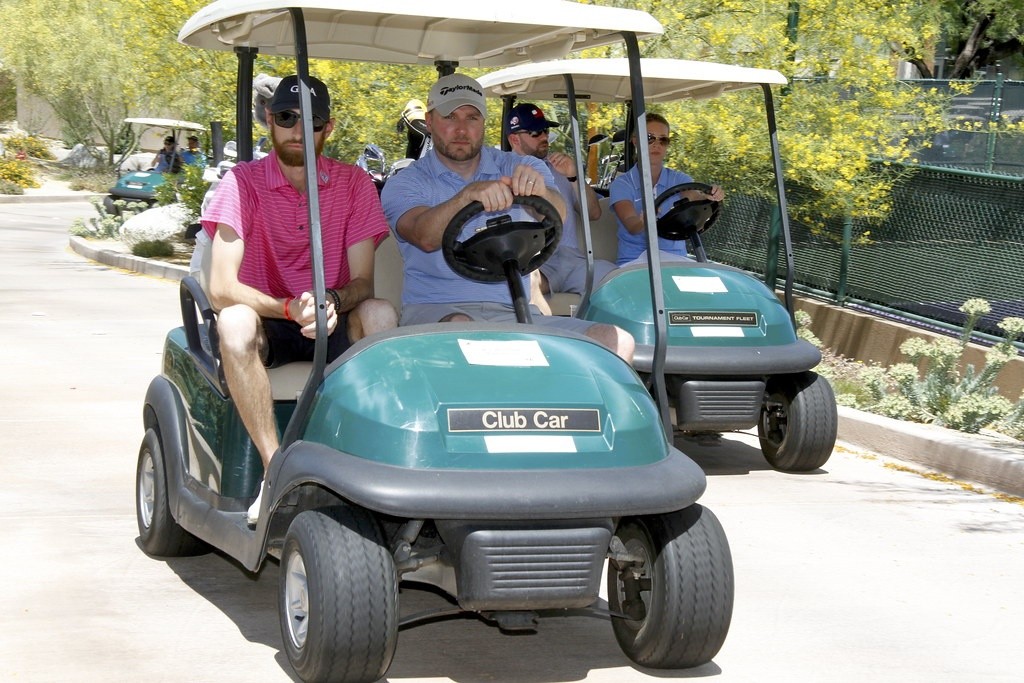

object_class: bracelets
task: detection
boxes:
[567,176,578,182]
[284,296,297,320]
[325,288,341,313]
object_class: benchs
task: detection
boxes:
[178,204,404,401]
[542,197,642,317]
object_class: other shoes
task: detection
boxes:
[247,480,265,527]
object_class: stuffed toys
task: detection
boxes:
[252,73,284,128]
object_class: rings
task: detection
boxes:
[527,179,534,185]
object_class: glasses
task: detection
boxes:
[275,111,329,132]
[165,142,173,145]
[633,133,670,146]
[512,127,550,137]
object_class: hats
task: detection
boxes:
[506,103,560,137]
[164,136,174,144]
[428,73,487,120]
[272,75,330,122]
[187,136,197,141]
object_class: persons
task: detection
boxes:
[505,103,620,298]
[609,114,726,268]
[179,136,207,175]
[200,74,397,530]
[380,73,635,367]
[152,136,184,174]
